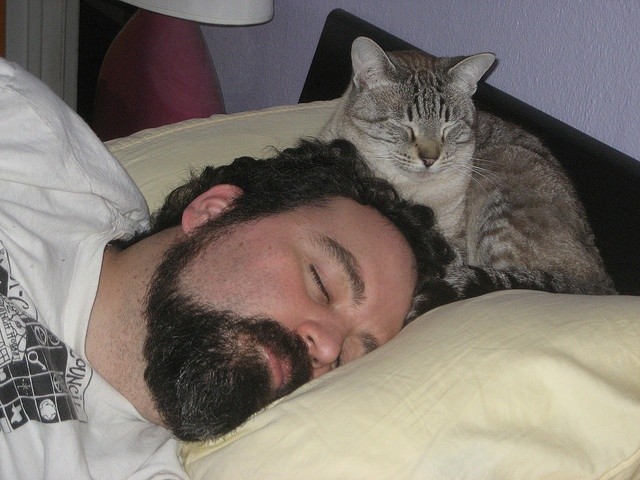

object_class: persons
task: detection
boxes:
[0,57,460,480]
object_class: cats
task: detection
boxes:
[314,33,620,328]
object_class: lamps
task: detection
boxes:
[92,1,276,147]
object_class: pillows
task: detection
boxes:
[94,94,640,480]
